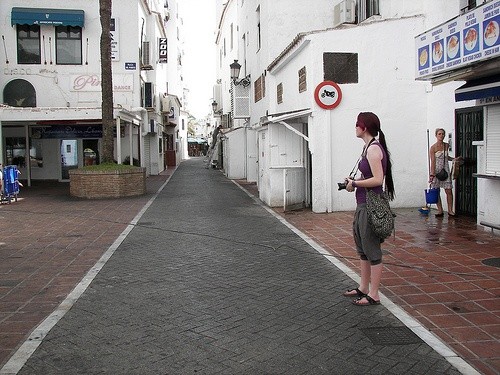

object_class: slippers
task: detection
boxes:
[343,287,367,297]
[351,295,380,305]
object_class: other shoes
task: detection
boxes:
[448,212,457,217]
[435,213,444,217]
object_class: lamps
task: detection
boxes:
[212,101,223,115]
[230,60,251,88]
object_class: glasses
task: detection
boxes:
[356,123,361,127]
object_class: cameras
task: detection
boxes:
[337,177,354,191]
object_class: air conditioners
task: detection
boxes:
[144,82,156,111]
[143,41,155,70]
[162,99,170,112]
[221,112,231,128]
[334,0,356,26]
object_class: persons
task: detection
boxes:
[342,112,395,304]
[429,128,461,218]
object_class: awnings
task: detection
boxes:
[11,7,84,27]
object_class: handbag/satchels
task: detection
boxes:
[365,189,396,238]
[436,169,449,181]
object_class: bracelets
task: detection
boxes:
[430,174,435,177]
[351,181,358,188]
